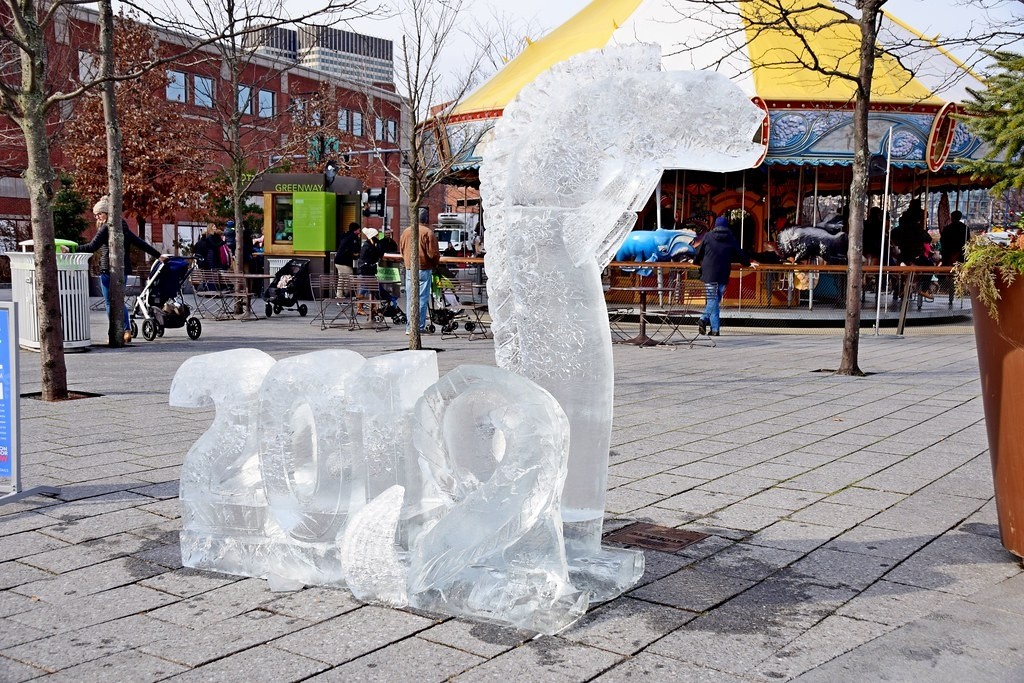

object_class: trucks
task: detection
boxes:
[432,211,483,258]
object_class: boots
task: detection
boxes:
[373,298,378,310]
[356,294,366,315]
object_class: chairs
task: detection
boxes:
[89,270,259,322]
[439,278,489,341]
[309,272,389,332]
[608,282,718,351]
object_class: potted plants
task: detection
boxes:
[949,47,1024,557]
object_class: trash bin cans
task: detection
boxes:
[4,239,91,353]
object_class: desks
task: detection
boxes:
[350,281,402,329]
[221,273,273,320]
[609,288,677,346]
[464,284,494,339]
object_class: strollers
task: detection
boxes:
[262,257,312,317]
[370,283,407,326]
[129,254,204,341]
[428,267,475,335]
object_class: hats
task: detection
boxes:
[715,217,728,228]
[92,195,108,214]
[361,228,378,239]
[418,208,427,222]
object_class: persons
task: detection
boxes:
[443,242,471,268]
[399,208,440,336]
[334,222,361,306]
[661,195,675,230]
[379,229,397,293]
[689,217,759,336]
[193,220,287,299]
[840,199,971,299]
[61,195,168,343]
[356,228,384,316]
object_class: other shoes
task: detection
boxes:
[406,332,410,335]
[419,331,430,335]
[708,330,719,336]
[124,331,132,343]
[698,318,706,335]
[919,290,933,298]
[899,291,904,297]
[337,302,350,305]
